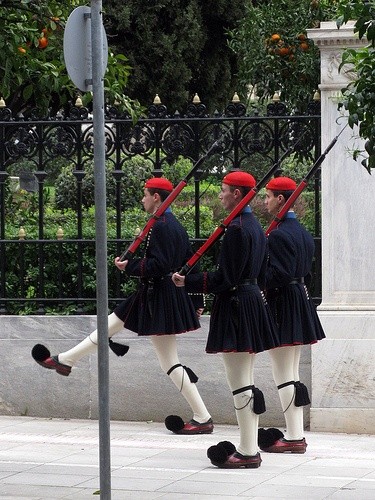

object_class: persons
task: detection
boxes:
[172,172,269,468]
[32,178,214,435]
[257,177,327,456]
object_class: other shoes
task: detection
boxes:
[258,427,308,455]
[207,441,262,469]
[165,414,215,435]
[31,343,73,377]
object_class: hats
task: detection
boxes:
[221,172,257,188]
[266,177,297,191]
[143,178,174,193]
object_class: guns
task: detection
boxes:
[116,129,233,265]
[177,122,317,277]
[263,112,352,239]
